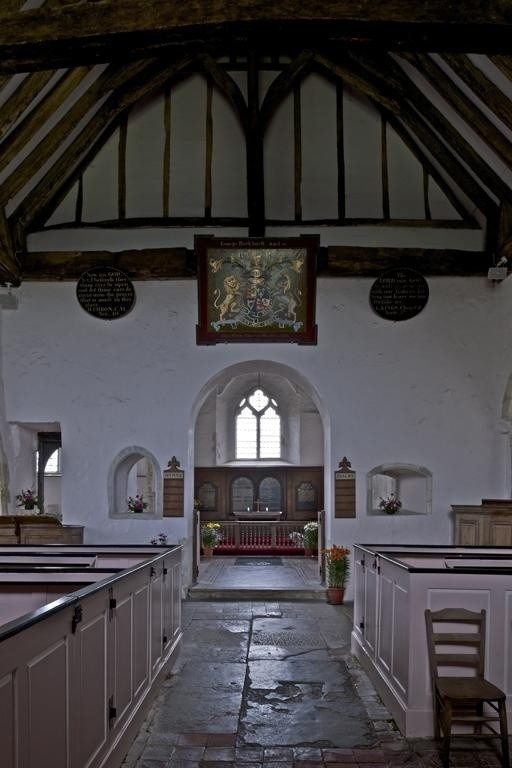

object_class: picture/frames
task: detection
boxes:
[197,237,317,343]
[387,510,396,514]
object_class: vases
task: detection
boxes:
[135,510,143,513]
[305,549,314,556]
[24,510,35,516]
[326,588,346,605]
[203,547,215,558]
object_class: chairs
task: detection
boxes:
[425,608,511,768]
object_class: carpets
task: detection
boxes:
[235,557,284,566]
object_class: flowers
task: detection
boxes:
[321,543,350,588]
[201,522,221,547]
[378,493,402,513]
[16,488,43,510]
[303,522,319,548]
[148,532,167,544]
[128,493,148,511]
